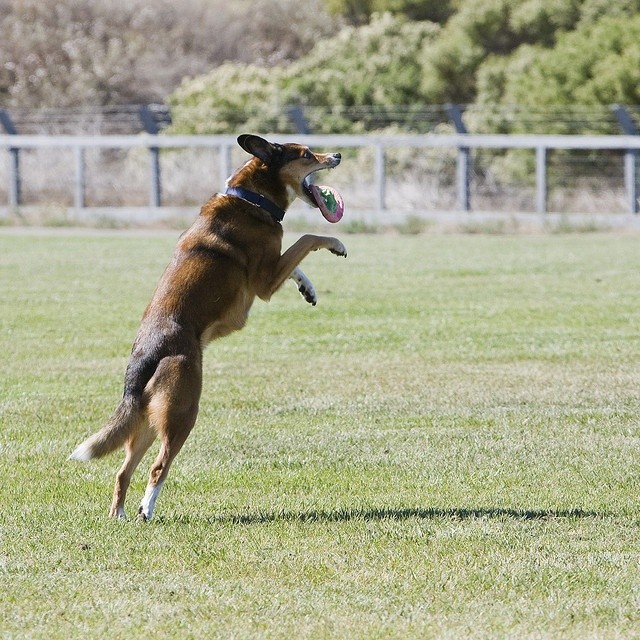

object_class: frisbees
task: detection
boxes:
[310,184,344,222]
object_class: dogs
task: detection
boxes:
[65,134,347,520]
